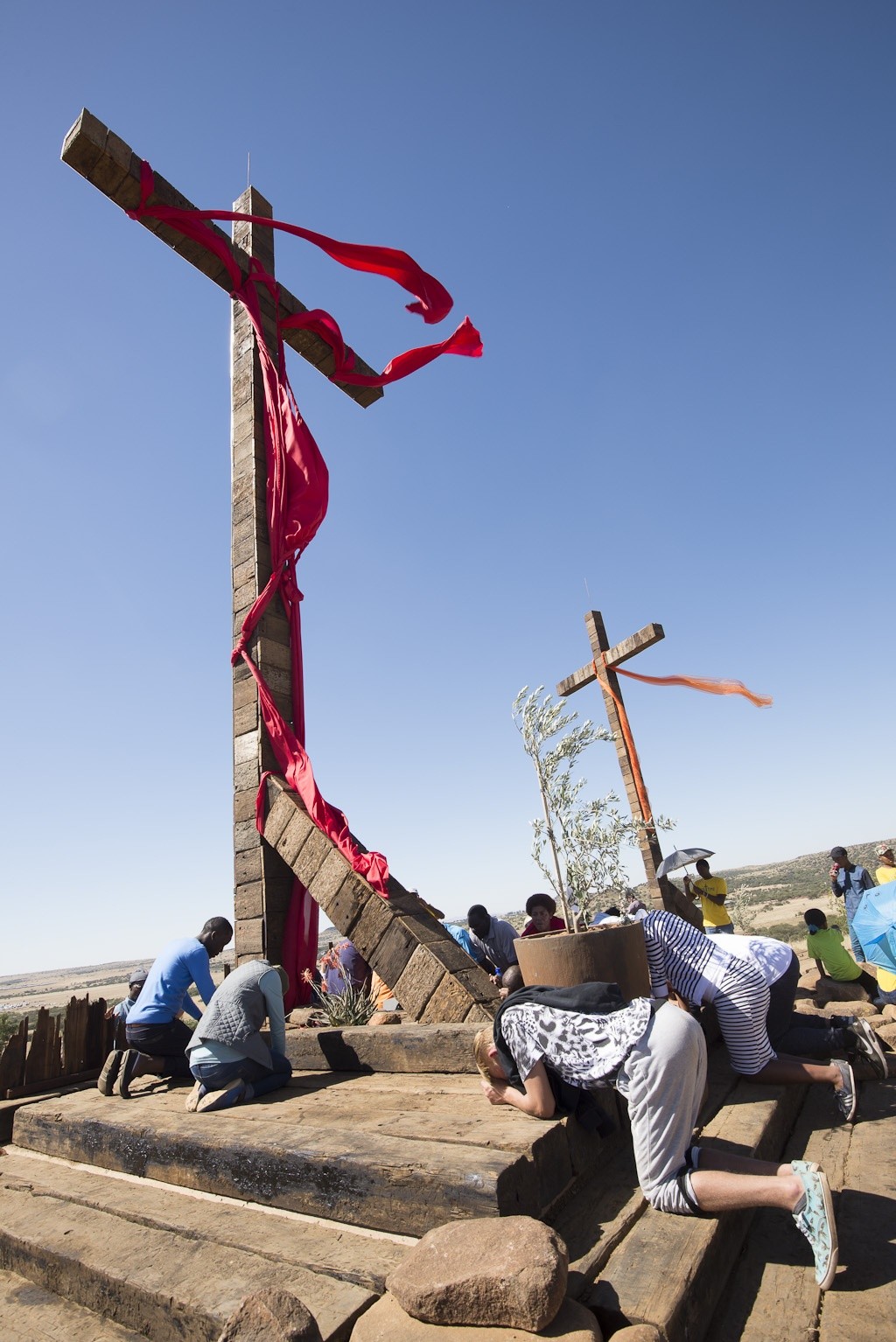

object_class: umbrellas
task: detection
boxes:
[655,845,716,901]
[852,880,896,974]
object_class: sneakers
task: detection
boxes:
[842,1015,861,1030]
[846,1018,889,1079]
[829,1058,856,1122]
[790,1170,839,1290]
[789,1159,821,1174]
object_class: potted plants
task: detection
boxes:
[511,683,676,1001]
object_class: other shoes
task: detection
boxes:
[184,1080,214,1113]
[112,1049,141,1099]
[97,1050,125,1096]
[195,1078,245,1114]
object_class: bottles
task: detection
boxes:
[831,863,839,877]
[495,967,503,988]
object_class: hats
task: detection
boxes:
[826,846,847,858]
[272,964,290,996]
[874,844,895,857]
[128,971,148,986]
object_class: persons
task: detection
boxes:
[876,966,896,1005]
[874,844,896,884]
[683,859,734,935]
[472,984,840,1289]
[103,968,185,1049]
[703,933,889,1080]
[319,938,404,1010]
[827,846,876,963]
[97,916,233,1099]
[597,909,856,1120]
[185,959,293,1112]
[804,908,884,1007]
[442,891,647,1002]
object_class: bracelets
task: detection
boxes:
[703,891,705,895]
[706,893,708,898]
